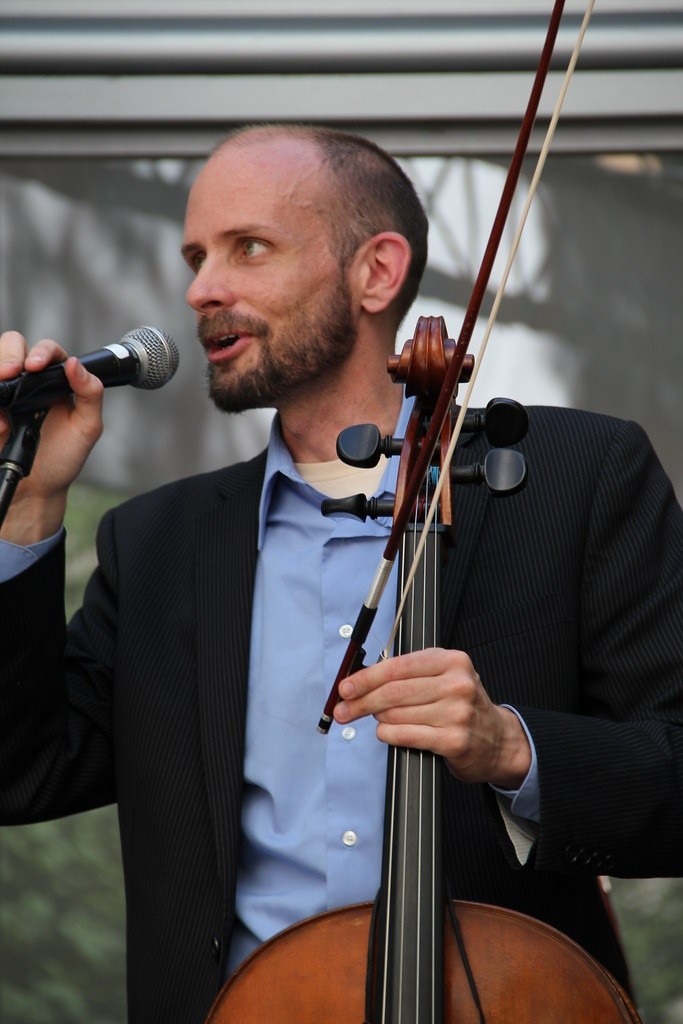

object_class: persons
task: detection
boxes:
[0,118,682,1024]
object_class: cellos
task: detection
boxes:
[202,316,646,1024]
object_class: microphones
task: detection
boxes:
[2,325,179,416]
